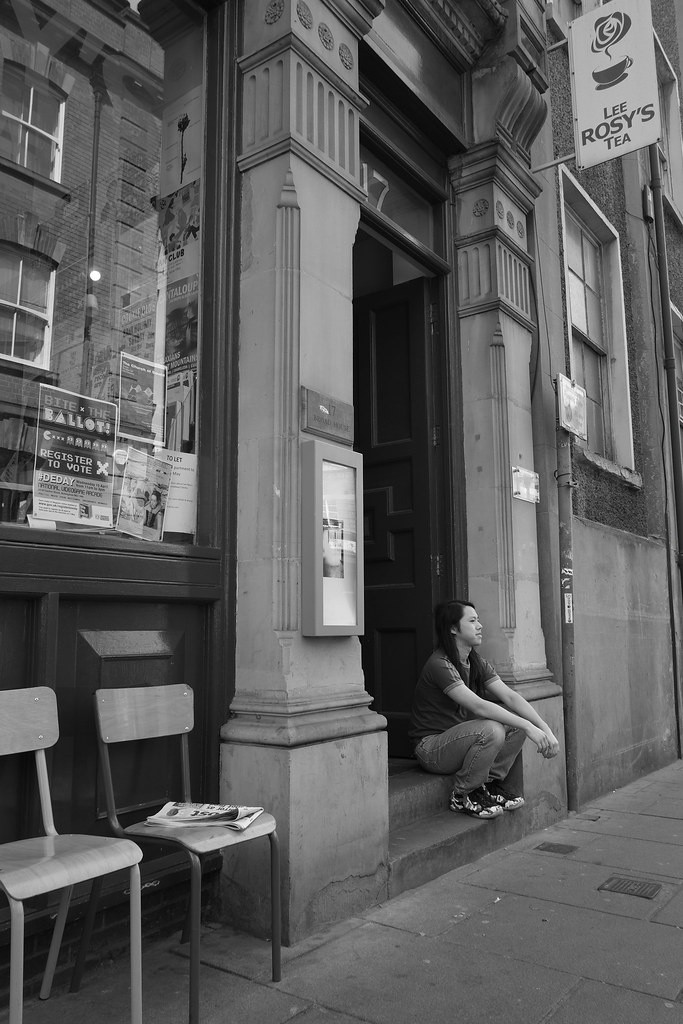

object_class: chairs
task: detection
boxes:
[0,686,143,1024]
[70,683,282,1024]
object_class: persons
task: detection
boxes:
[143,491,163,540]
[408,600,560,820]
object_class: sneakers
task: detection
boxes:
[481,777,525,812]
[448,786,504,819]
